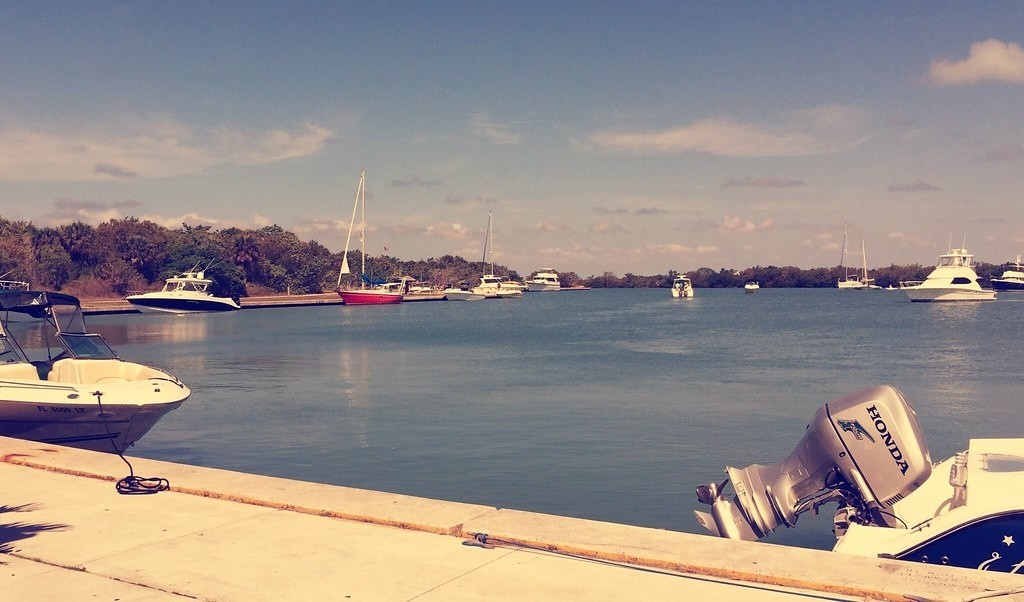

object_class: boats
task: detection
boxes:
[445,281,486,301]
[899,231,999,300]
[125,257,242,315]
[497,290,524,299]
[0,279,191,452]
[692,384,1024,575]
[869,284,882,290]
[886,288,898,292]
[671,274,694,298]
[744,282,761,294]
[375,275,444,301]
[989,254,1024,288]
[524,268,560,293]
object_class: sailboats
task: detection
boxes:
[837,238,869,290]
[469,211,527,295]
[335,168,405,305]
